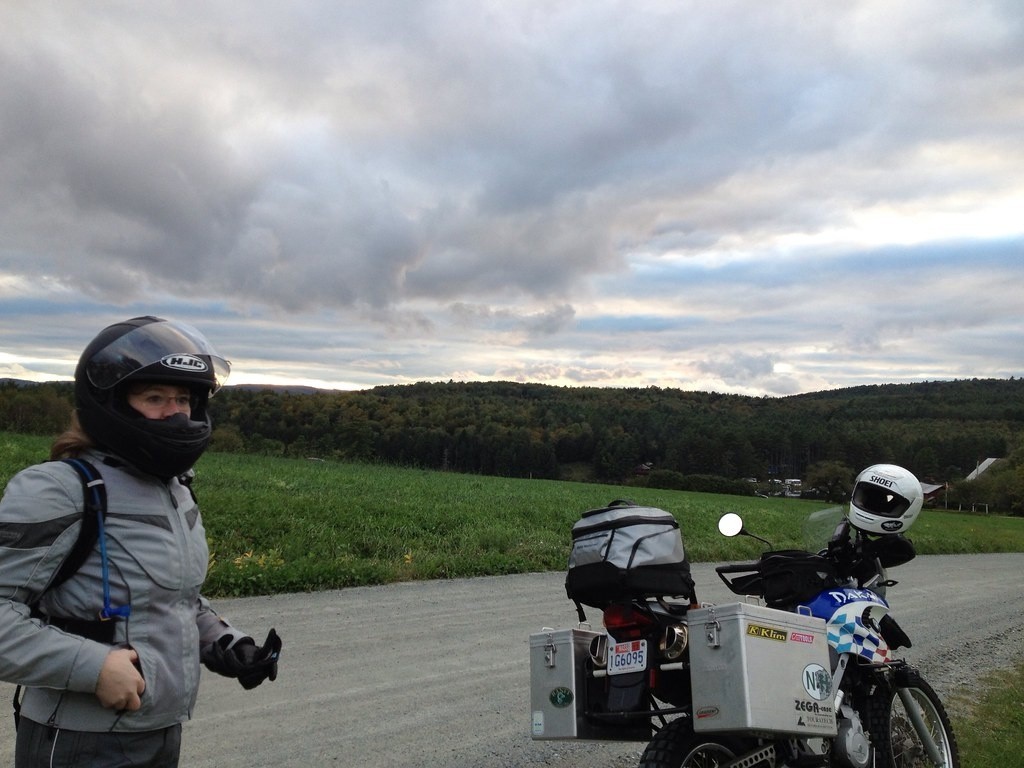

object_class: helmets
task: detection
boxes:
[849,463,925,537]
[71,314,232,482]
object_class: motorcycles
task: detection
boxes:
[529,464,961,768]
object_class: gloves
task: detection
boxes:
[206,628,282,690]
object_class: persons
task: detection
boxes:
[0,313,278,768]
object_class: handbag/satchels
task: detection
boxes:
[565,499,702,604]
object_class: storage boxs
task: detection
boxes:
[528,621,654,742]
[686,602,840,738]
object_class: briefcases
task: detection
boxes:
[687,602,838,735]
[529,622,604,740]
